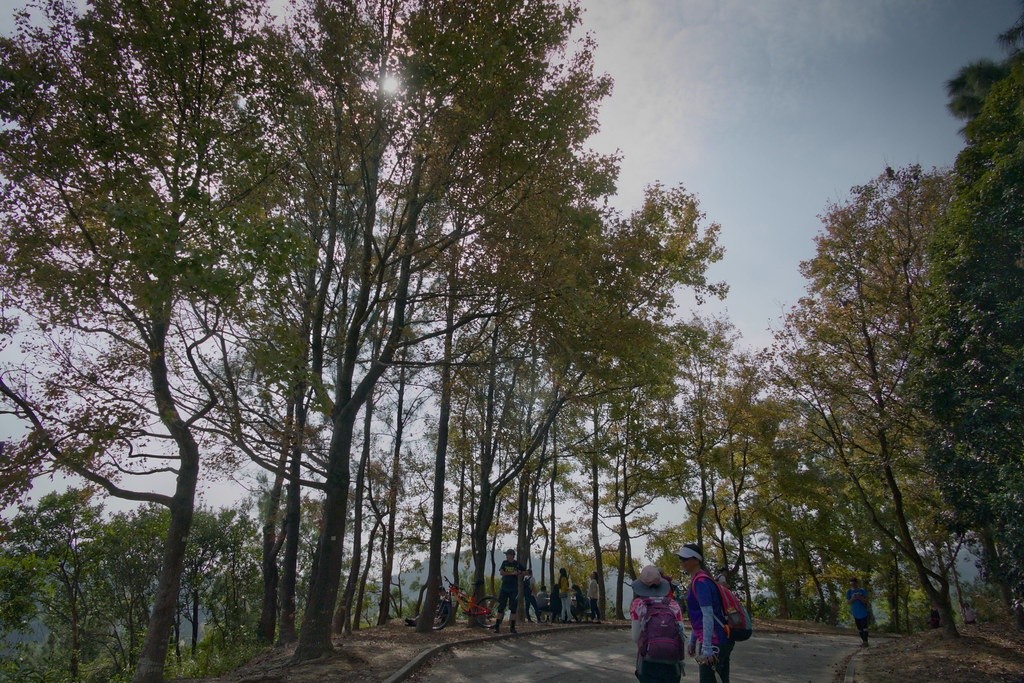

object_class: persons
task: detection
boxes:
[847,578,868,647]
[403,549,601,633]
[926,602,975,630]
[629,544,734,683]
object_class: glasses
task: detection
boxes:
[679,556,693,561]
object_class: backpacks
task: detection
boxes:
[577,593,590,608]
[638,597,688,664]
[524,577,532,596]
[715,575,724,582]
[692,573,752,641]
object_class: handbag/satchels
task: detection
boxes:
[559,593,568,598]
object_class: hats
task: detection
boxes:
[560,568,566,575]
[631,565,670,597]
[503,549,515,555]
[676,547,702,562]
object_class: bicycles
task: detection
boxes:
[433,576,504,630]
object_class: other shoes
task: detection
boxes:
[405,618,416,627]
[537,620,544,623]
[510,629,517,633]
[596,622,601,624]
[862,642,868,647]
[589,621,593,623]
[494,630,499,633]
[528,620,535,623]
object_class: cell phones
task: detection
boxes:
[695,655,707,666]
